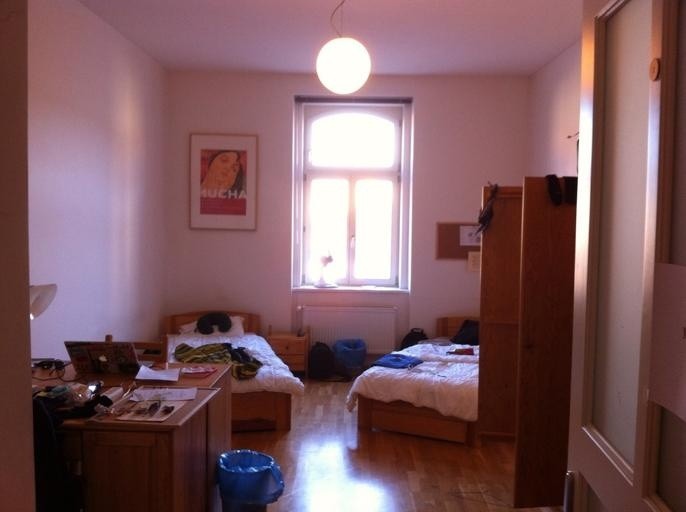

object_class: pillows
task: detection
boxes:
[452,319,480,346]
[189,316,244,338]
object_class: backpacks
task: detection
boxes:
[399,328,429,351]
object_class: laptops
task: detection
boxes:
[64,341,155,374]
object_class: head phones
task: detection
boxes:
[33,360,65,381]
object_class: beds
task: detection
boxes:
[156,312,293,434]
[355,315,480,447]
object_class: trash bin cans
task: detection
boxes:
[336,340,365,378]
[217,450,275,512]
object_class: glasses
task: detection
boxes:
[34,360,65,370]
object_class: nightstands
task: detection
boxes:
[272,334,306,373]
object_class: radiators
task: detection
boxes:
[300,303,397,355]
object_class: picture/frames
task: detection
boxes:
[434,222,483,263]
[189,131,258,231]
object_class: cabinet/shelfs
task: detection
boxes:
[475,177,574,509]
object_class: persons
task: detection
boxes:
[199,150,243,201]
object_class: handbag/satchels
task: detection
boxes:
[309,341,334,379]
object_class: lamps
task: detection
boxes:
[315,0,371,95]
[30,282,60,322]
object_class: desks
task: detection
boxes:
[32,358,232,512]
